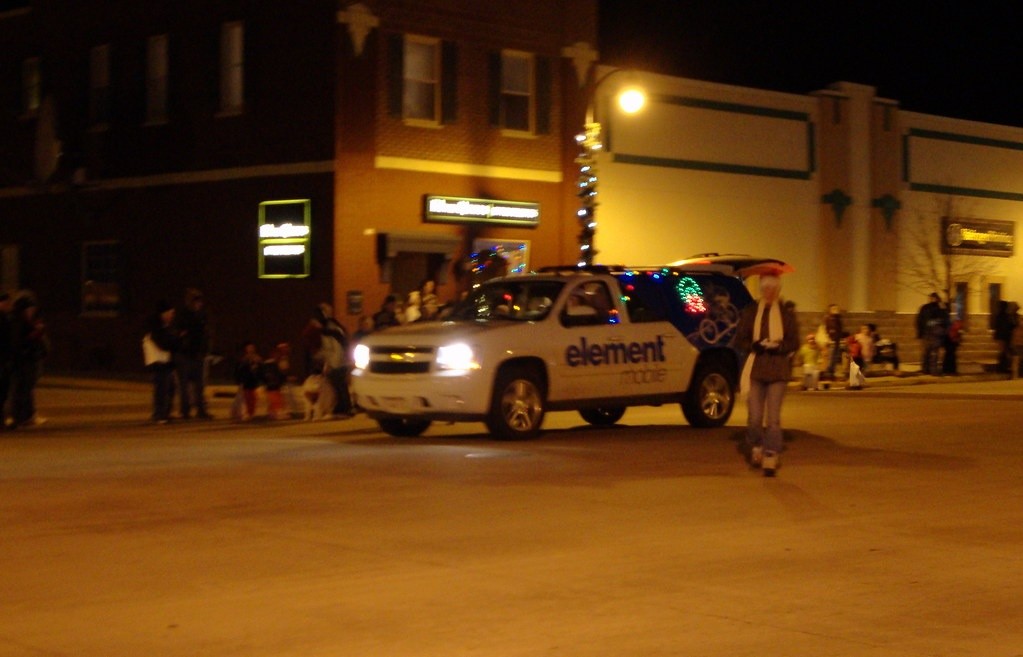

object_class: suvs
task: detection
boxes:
[348,253,761,442]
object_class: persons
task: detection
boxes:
[797,288,1023,401]
[733,274,801,475]
[1,245,528,441]
[562,283,597,317]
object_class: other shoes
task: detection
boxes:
[752,445,764,467]
[762,453,779,476]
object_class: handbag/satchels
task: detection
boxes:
[144,333,171,366]
